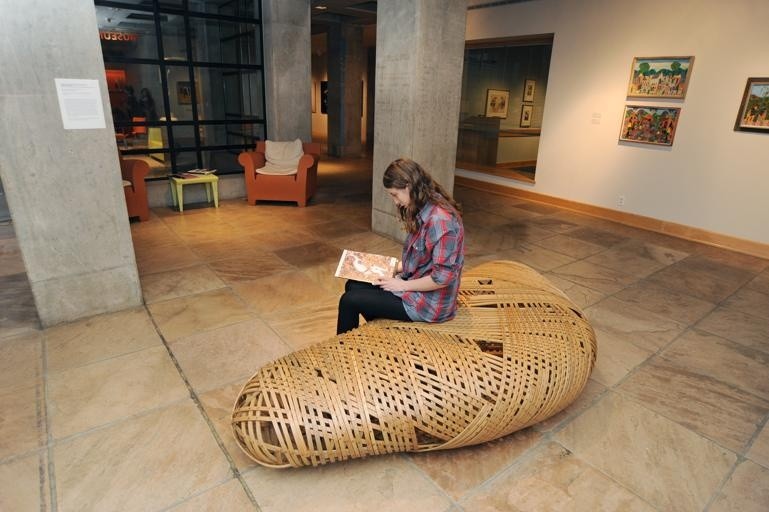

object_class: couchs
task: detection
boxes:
[116,149,151,225]
[236,138,323,208]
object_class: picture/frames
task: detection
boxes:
[523,80,536,103]
[619,104,681,147]
[733,77,769,133]
[626,55,696,99]
[484,89,510,119]
[519,104,534,128]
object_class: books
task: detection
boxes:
[171,168,217,180]
[334,248,398,287]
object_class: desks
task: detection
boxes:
[168,173,220,211]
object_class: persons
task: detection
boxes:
[499,96,505,113]
[336,160,465,335]
[490,97,498,111]
[525,112,528,120]
[527,82,532,95]
[750,104,760,125]
[123,85,140,120]
[138,89,159,121]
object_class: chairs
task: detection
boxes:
[130,116,147,147]
